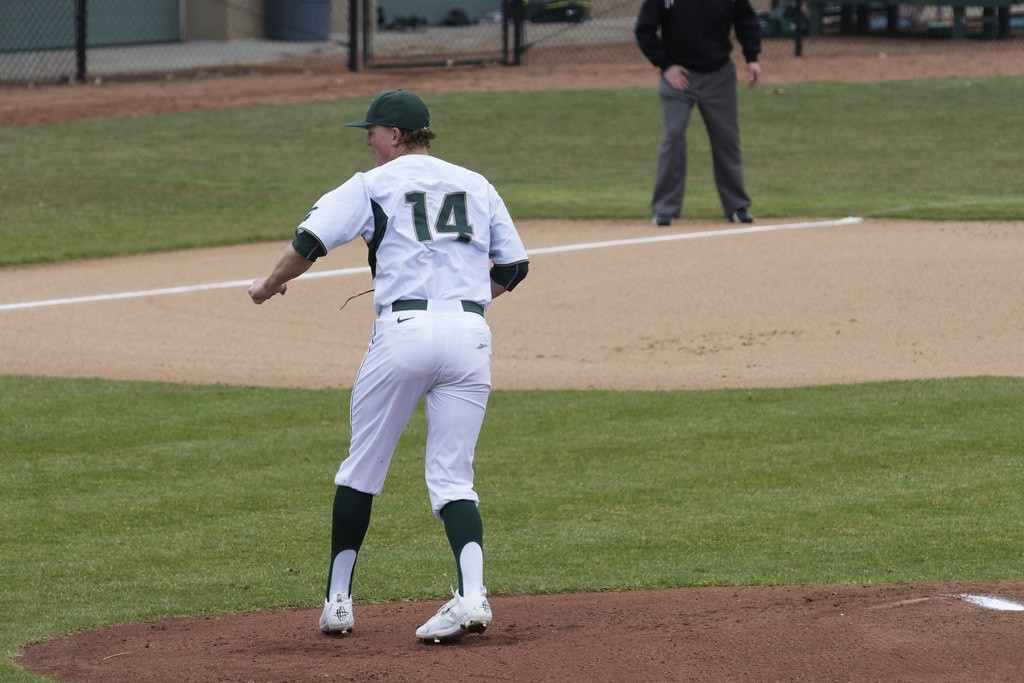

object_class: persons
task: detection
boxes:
[246,86,532,644]
[631,0,766,226]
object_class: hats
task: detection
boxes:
[345,88,430,128]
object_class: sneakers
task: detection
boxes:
[730,210,753,223]
[415,589,493,643]
[318,596,356,635]
[657,215,674,225]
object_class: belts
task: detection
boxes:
[390,299,484,317]
